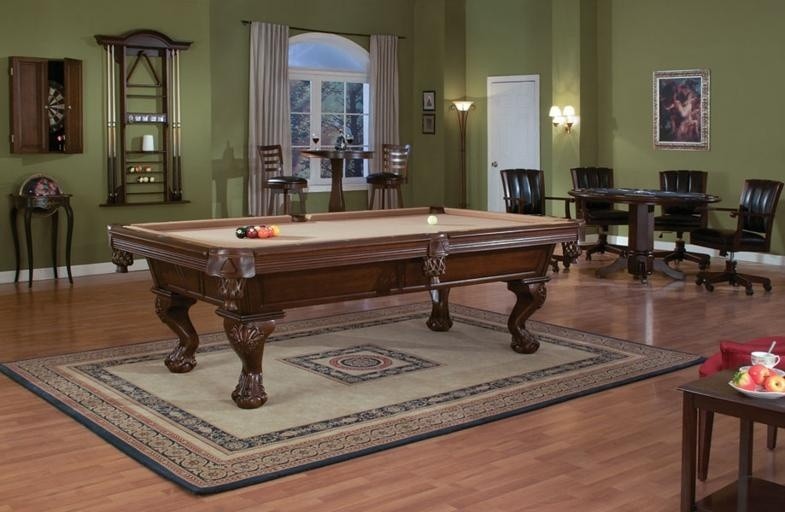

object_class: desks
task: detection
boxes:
[107,206,585,410]
[675,366,785,511]
[300,149,374,213]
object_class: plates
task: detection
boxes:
[17,173,61,218]
[729,365,785,399]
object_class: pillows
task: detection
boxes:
[719,340,785,369]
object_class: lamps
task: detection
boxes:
[548,104,581,134]
[449,99,476,209]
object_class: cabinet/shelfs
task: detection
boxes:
[8,55,83,155]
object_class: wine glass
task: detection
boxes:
[347,134,354,151]
[311,133,321,152]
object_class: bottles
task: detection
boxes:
[143,135,154,151]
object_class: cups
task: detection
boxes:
[750,351,781,369]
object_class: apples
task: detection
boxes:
[748,364,770,385]
[765,376,785,393]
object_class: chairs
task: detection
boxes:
[500,166,784,296]
[256,144,308,216]
[699,335,785,482]
[366,143,412,210]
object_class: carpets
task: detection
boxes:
[1,300,707,494]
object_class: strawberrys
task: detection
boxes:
[735,372,756,390]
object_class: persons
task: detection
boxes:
[662,82,701,142]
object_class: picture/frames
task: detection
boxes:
[422,90,436,111]
[652,68,712,152]
[422,114,435,134]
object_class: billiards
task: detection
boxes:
[428,215,437,224]
[236,224,281,239]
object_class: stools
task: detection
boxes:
[8,193,74,288]
